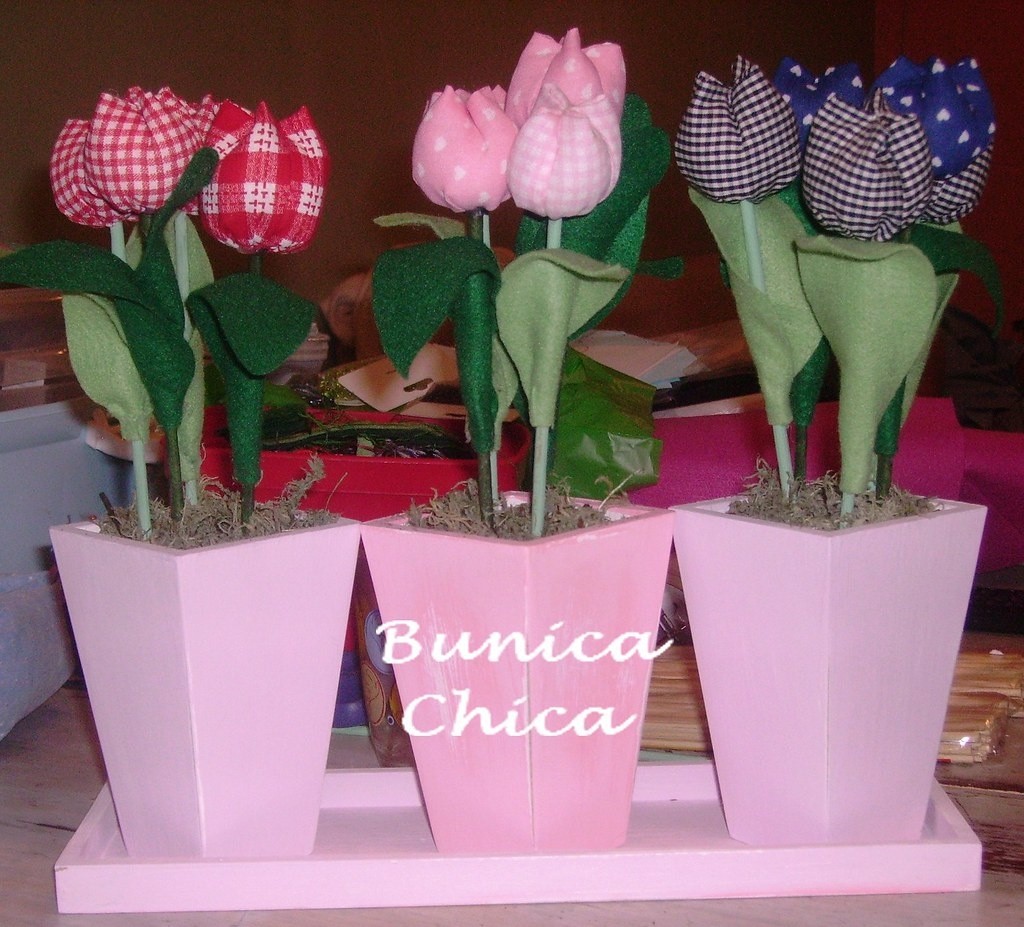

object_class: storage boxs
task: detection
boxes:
[160,409,526,648]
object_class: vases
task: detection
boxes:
[680,481,990,842]
[37,497,362,866]
[359,491,677,853]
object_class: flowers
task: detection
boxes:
[669,54,997,508]
[0,82,327,525]
[413,27,651,529]
